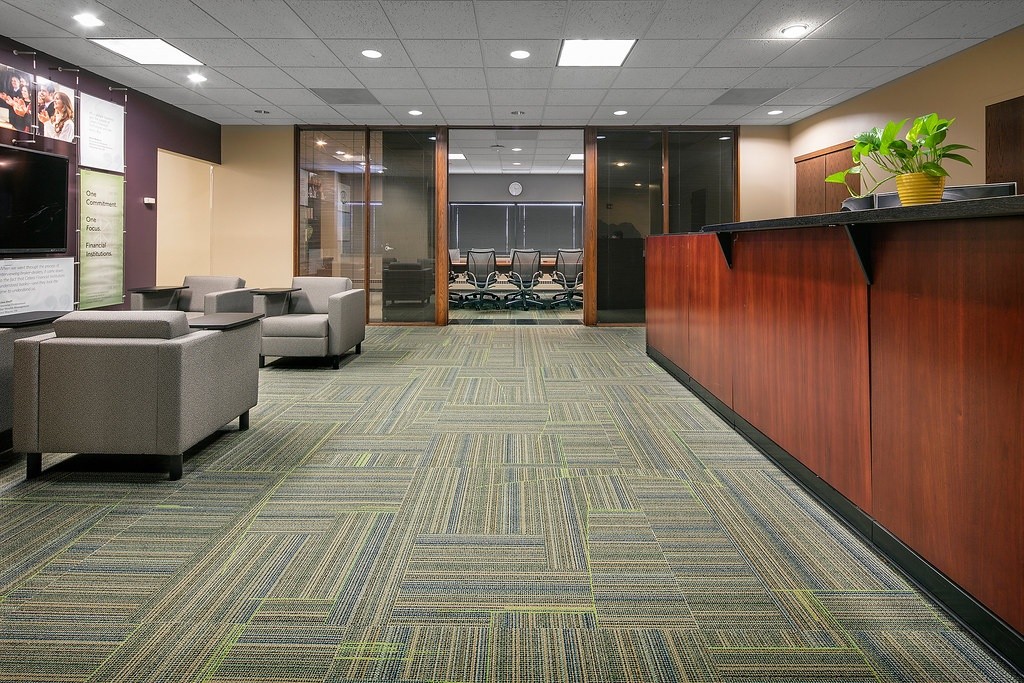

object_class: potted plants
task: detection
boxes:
[825,113,978,206]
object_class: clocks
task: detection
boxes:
[508,181,523,196]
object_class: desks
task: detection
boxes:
[0,311,71,328]
[250,287,302,315]
[187,312,265,330]
[127,285,189,294]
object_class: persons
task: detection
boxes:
[36,80,75,142]
[0,73,33,133]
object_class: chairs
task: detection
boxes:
[448,248,501,311]
[550,248,583,311]
[504,249,547,311]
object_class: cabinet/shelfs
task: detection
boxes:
[320,171,361,259]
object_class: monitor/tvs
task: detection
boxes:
[873,182,1017,208]
[0,143,69,253]
[840,194,876,212]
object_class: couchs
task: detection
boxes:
[131,275,260,320]
[0,310,76,433]
[12,309,265,480]
[383,262,432,308]
[249,277,366,371]
